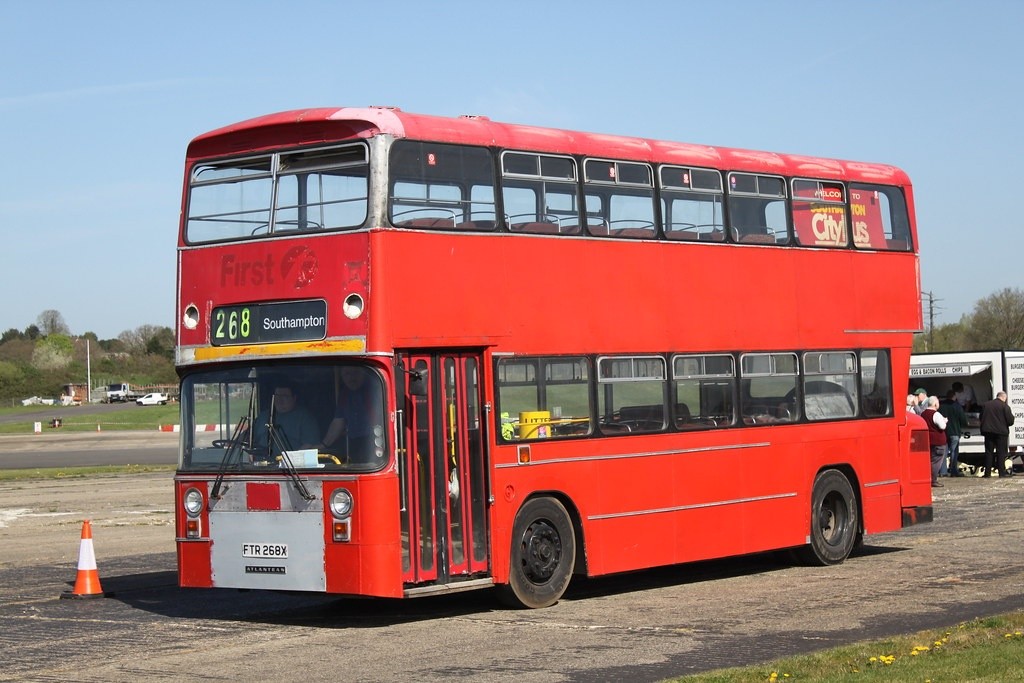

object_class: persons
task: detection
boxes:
[940,390,967,477]
[313,368,375,464]
[921,396,947,488]
[913,388,929,415]
[223,384,320,461]
[952,382,976,412]
[979,391,1015,477]
[907,395,915,414]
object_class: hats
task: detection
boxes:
[912,388,927,395]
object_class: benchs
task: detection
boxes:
[251,220,320,236]
[390,208,801,247]
[570,406,790,435]
[883,232,909,250]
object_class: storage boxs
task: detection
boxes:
[965,412,980,426]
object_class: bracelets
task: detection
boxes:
[321,442,328,448]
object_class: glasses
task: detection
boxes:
[275,393,293,400]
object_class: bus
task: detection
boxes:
[173,107,932,616]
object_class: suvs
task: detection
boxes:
[136,393,168,406]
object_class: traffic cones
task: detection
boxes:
[60,520,115,599]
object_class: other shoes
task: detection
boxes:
[932,481,944,487]
[951,473,965,477]
[999,473,1012,478]
[982,474,991,478]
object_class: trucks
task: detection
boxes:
[105,382,146,403]
[911,351,1024,464]
